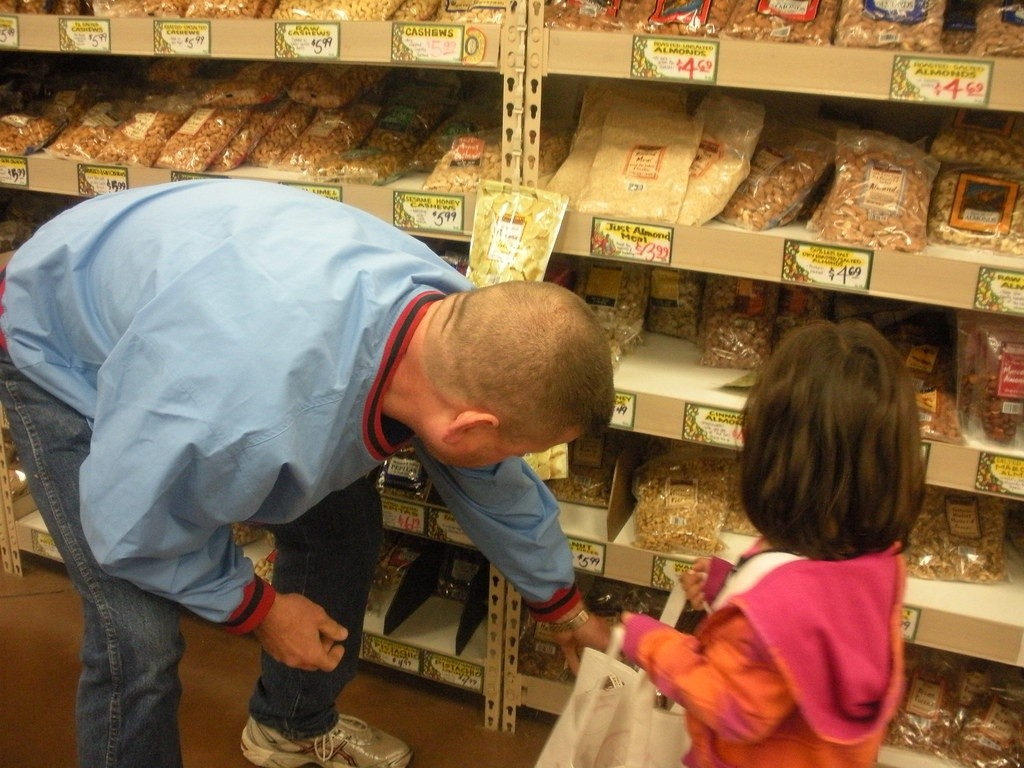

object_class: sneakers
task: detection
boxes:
[239,712,413,768]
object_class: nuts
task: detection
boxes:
[542,0,1024,60]
[708,119,940,254]
[0,0,580,191]
[226,521,276,586]
[572,261,1024,450]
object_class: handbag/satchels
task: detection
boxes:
[536,571,690,768]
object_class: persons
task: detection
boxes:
[614,319,926,768]
[0,177,614,768]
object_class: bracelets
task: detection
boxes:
[540,609,590,633]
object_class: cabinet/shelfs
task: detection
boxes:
[501,0,1024,768]
[1,1,529,730]
